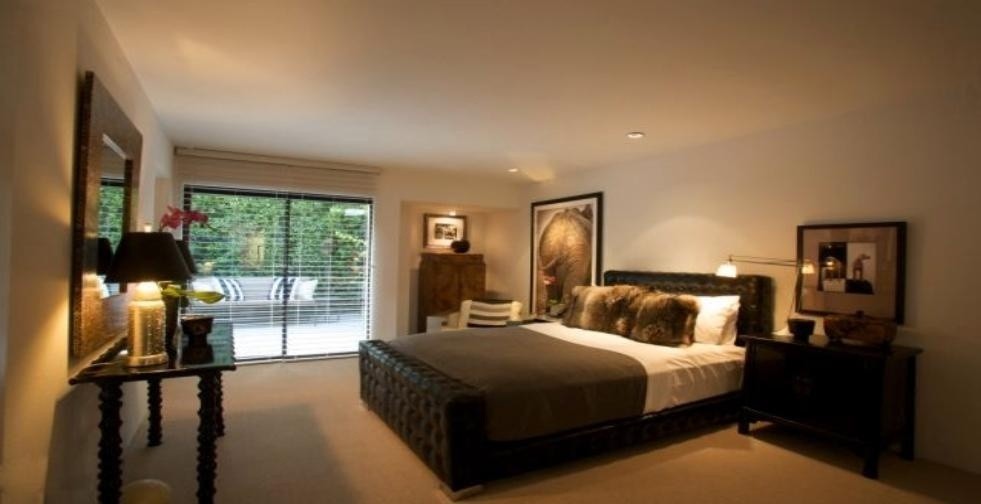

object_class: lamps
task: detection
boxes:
[103,230,188,365]
[96,235,121,300]
[715,252,806,337]
[161,237,193,340]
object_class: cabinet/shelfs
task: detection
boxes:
[737,328,924,486]
[417,254,486,334]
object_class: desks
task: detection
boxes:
[65,316,237,503]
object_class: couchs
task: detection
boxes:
[194,268,319,327]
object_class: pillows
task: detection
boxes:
[193,276,225,306]
[722,316,738,346]
[622,291,698,344]
[287,272,318,301]
[671,292,738,350]
[560,279,633,336]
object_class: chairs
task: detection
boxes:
[423,290,526,332]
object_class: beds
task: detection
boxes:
[345,265,776,500]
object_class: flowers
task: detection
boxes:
[157,203,214,316]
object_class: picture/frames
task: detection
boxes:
[422,212,468,248]
[528,190,606,321]
[794,221,907,320]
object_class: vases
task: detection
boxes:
[179,313,213,340]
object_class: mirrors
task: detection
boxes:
[65,69,145,360]
